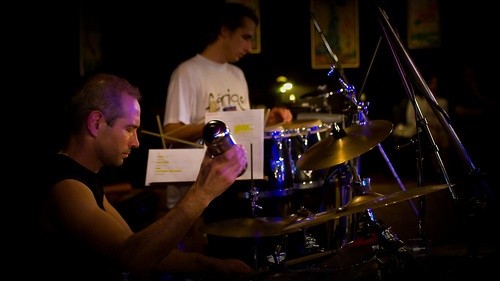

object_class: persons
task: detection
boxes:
[22,73,254,281]
[164,2,292,143]
[406,75,449,151]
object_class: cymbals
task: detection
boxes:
[206,214,303,238]
[296,120,394,172]
[283,183,454,233]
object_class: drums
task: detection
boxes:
[253,121,406,281]
